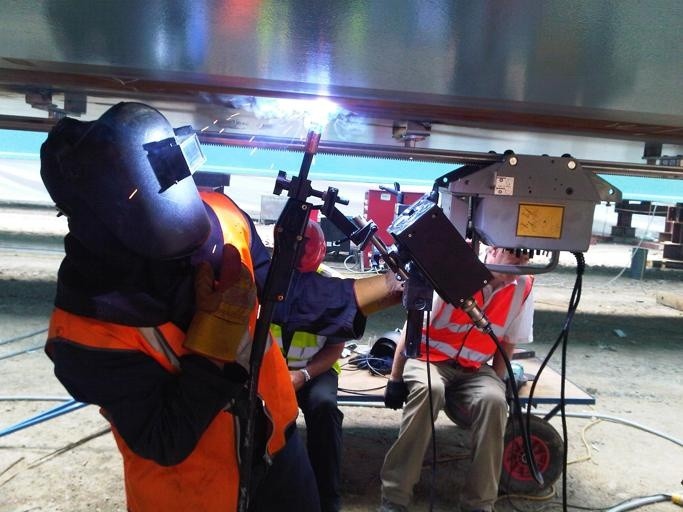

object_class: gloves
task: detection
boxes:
[384,380,410,410]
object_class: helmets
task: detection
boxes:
[297,219,326,272]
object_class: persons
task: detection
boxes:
[376,241,537,510]
[242,216,346,511]
[35,100,412,510]
